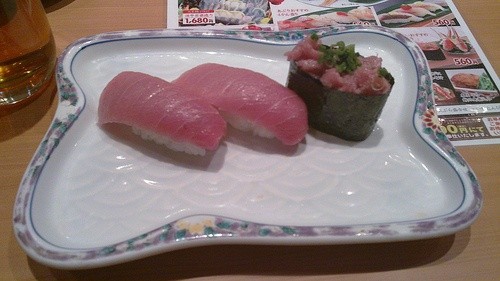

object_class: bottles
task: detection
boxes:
[0,0,57,106]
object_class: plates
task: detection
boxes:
[444,69,495,92]
[11,25,484,268]
[438,37,475,54]
[377,0,453,27]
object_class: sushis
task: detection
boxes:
[96,70,227,157]
[283,32,395,140]
[171,63,308,145]
